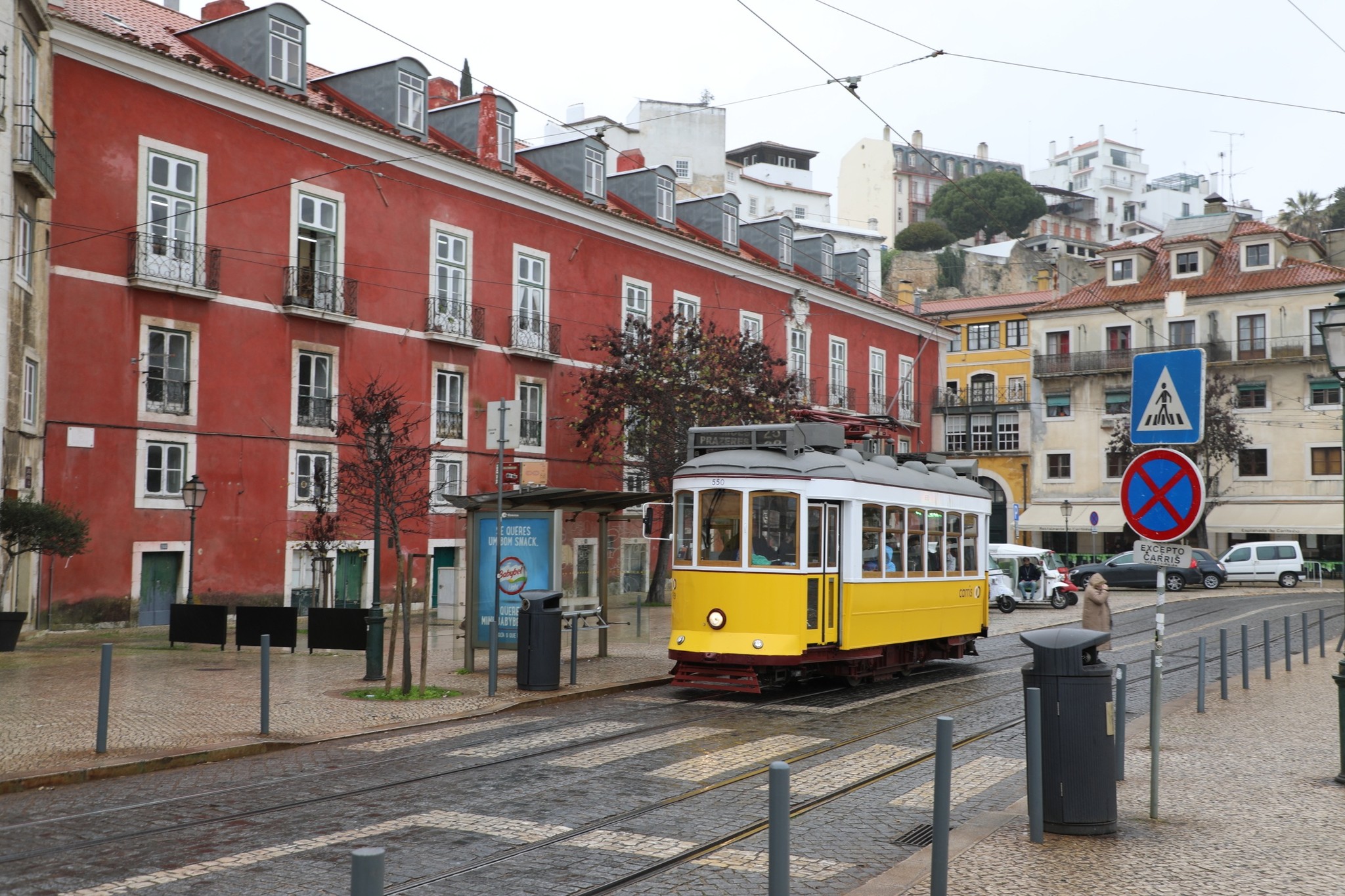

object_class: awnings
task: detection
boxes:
[1009,504,1345,535]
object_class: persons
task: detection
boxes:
[777,511,833,561]
[1017,558,1041,601]
[1082,573,1113,665]
[862,532,970,572]
[718,518,781,565]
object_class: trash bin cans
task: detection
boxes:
[1015,626,1117,834]
[516,591,563,690]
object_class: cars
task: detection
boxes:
[1190,547,1229,589]
[1068,549,1204,593]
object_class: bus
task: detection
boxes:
[642,423,993,691]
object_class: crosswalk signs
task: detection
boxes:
[1130,347,1206,445]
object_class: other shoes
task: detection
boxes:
[1022,598,1026,602]
[1030,597,1033,602]
[1096,659,1105,664]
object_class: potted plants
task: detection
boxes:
[0,488,92,652]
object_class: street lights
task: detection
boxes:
[363,410,395,684]
[1315,289,1345,785]
[183,474,207,608]
[1060,499,1073,568]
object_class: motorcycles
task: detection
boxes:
[988,543,1080,614]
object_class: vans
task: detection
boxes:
[1216,540,1305,588]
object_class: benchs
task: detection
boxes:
[561,610,610,632]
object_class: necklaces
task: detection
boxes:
[1025,567,1030,579]
